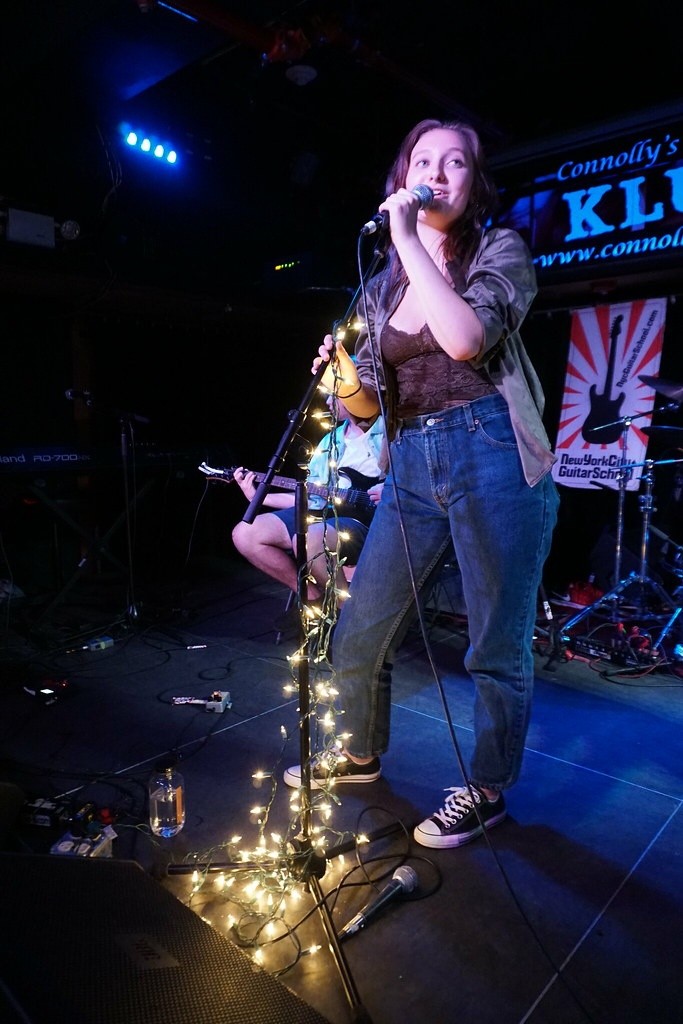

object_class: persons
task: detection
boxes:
[283,120,559,849]
[232,385,385,616]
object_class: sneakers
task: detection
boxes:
[284,747,380,789]
[414,783,506,848]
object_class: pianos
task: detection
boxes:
[0,449,221,484]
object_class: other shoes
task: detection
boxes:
[308,593,341,663]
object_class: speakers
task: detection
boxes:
[0,846,328,1024]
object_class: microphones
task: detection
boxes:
[336,866,421,944]
[361,183,434,235]
[65,388,90,401]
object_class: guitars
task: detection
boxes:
[194,460,385,526]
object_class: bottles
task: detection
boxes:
[147,760,186,838]
[24,795,113,827]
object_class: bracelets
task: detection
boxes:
[335,379,362,399]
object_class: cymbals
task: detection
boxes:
[637,370,683,417]
[639,425,683,446]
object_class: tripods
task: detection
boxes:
[166,225,426,1023]
[553,399,683,663]
[52,401,193,647]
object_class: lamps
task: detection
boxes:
[0,206,81,252]
